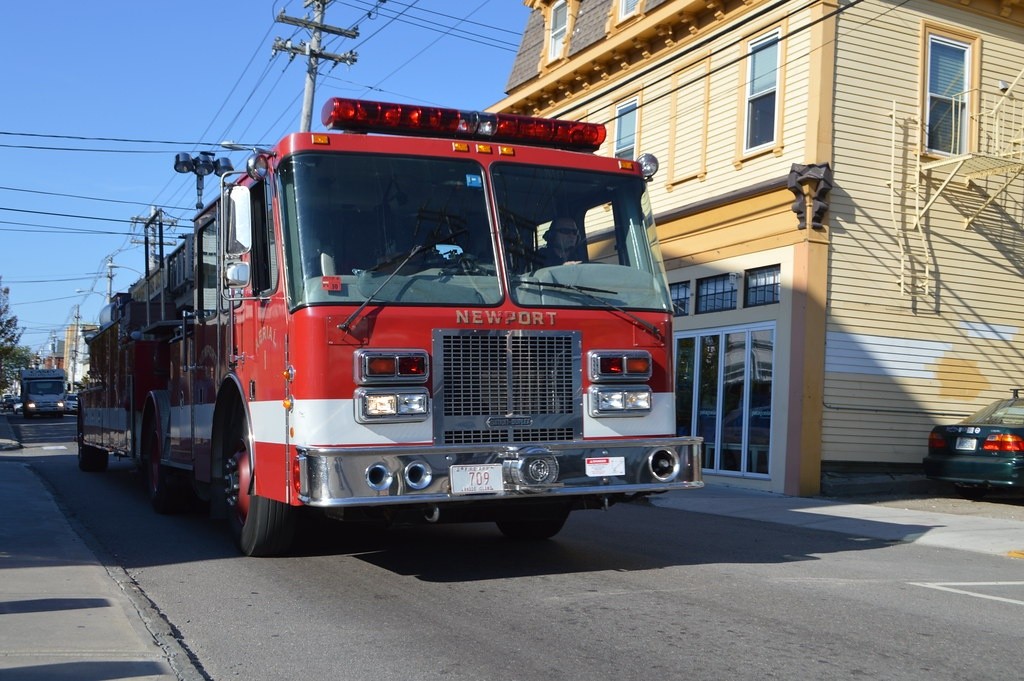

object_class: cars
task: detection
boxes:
[2,394,23,414]
[65,393,78,414]
[923,387,1024,506]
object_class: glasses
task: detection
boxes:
[553,228,579,237]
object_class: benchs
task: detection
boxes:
[702,442,769,473]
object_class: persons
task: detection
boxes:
[532,216,584,269]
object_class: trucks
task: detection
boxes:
[20,368,67,419]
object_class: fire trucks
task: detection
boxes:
[75,97,705,561]
